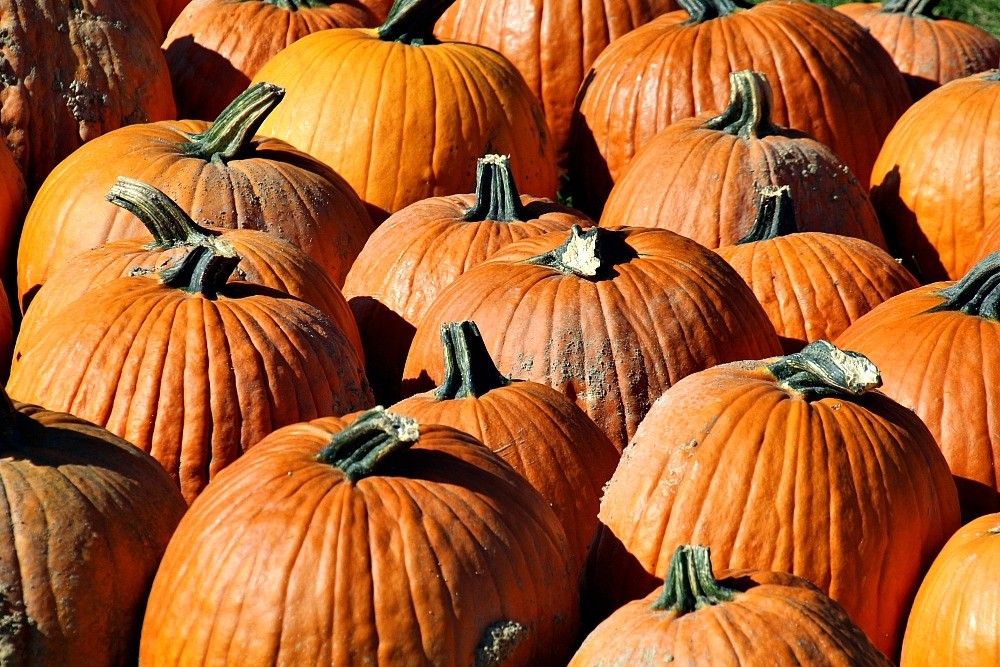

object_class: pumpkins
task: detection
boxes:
[0,0,1000,667]
